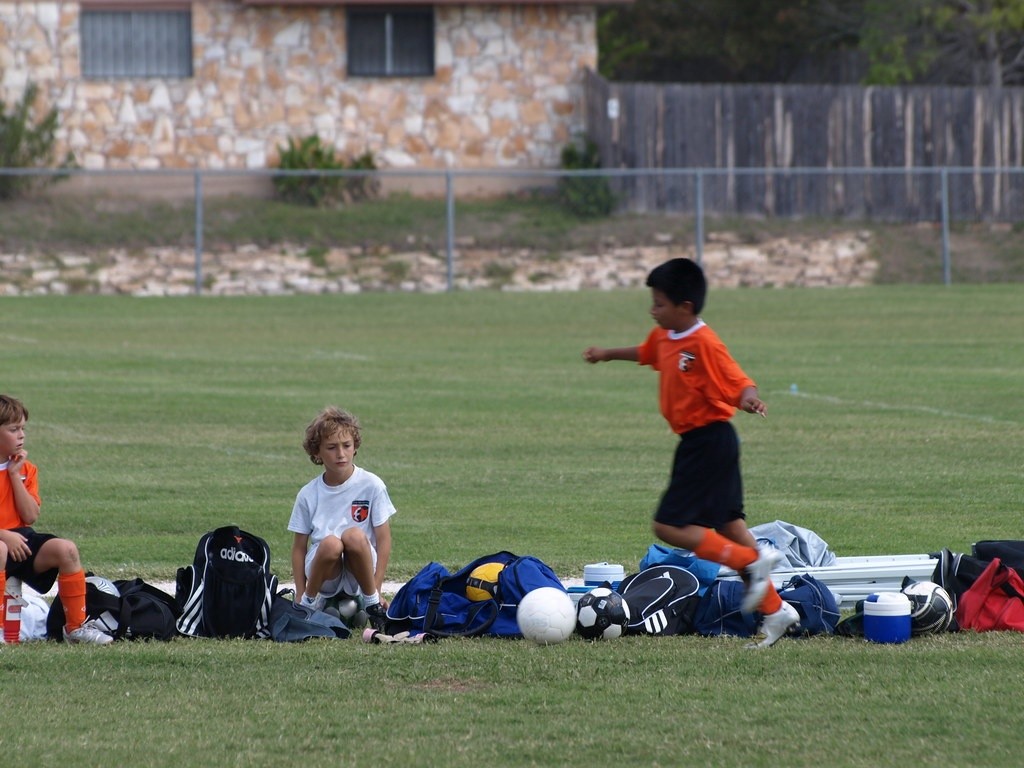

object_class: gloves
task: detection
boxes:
[362,628,436,645]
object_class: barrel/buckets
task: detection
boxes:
[583,562,624,591]
[567,586,598,604]
[863,592,911,642]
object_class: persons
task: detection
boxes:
[585,258,800,651]
[1,393,115,646]
[284,407,397,632]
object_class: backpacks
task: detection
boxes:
[175,526,279,641]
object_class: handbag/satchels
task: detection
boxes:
[268,588,352,643]
[46,572,175,643]
[598,565,699,637]
[693,573,841,641]
[833,575,917,638]
[927,540,1024,634]
[18,594,50,641]
[385,551,567,638]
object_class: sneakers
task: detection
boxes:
[62,615,113,645]
[362,593,386,635]
[743,600,800,650]
[739,544,777,614]
[300,592,326,611]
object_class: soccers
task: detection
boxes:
[516,586,577,645]
[575,587,631,641]
[85,576,120,598]
[322,590,368,631]
[900,580,954,637]
[465,562,506,602]
